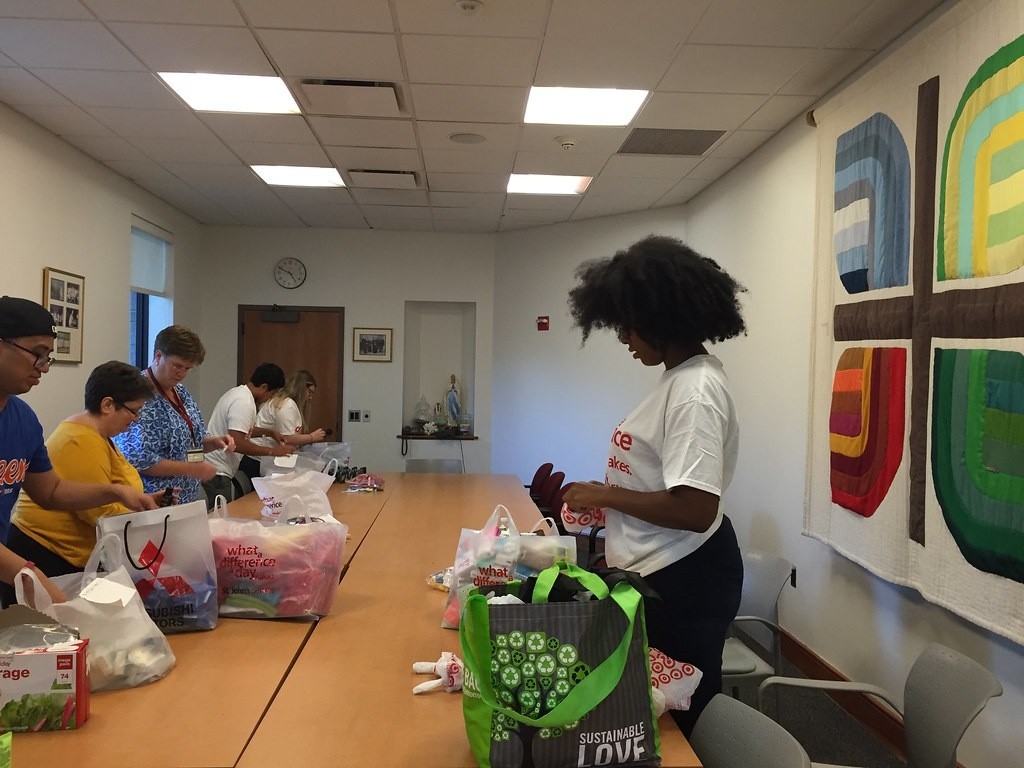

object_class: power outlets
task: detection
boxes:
[363,410,371,422]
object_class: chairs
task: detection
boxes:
[690,547,1004,768]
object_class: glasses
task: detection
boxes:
[0,337,55,369]
[112,396,143,418]
[162,351,194,374]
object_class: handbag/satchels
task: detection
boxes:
[96,499,218,633]
[251,459,341,525]
[459,561,663,768]
[439,505,577,630]
[260,434,329,476]
[304,441,351,468]
[207,494,348,621]
[13,534,177,693]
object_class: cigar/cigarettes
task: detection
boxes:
[221,445,228,453]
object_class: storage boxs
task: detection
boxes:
[0,605,90,732]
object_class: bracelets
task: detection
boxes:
[310,434,313,441]
[11,562,35,588]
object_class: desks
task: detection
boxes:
[11,471,704,768]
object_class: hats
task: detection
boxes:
[0,295,57,338]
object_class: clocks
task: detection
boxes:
[274,257,307,289]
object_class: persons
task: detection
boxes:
[359,335,384,354]
[107,326,235,509]
[199,362,296,511]
[444,373,461,429]
[562,235,745,741]
[0,295,159,612]
[238,370,325,492]
[50,279,81,327]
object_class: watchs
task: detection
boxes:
[0,361,180,611]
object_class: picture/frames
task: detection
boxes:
[44,267,85,363]
[353,327,393,362]
